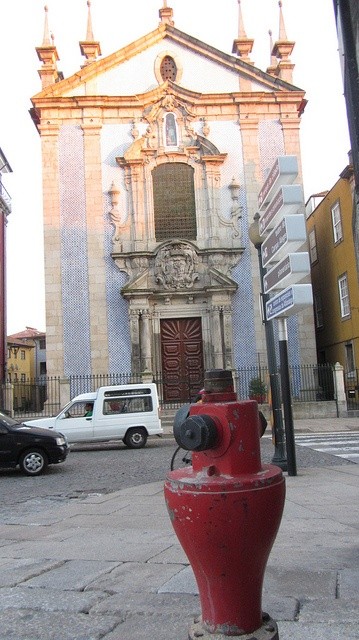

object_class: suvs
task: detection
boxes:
[19,384,162,449]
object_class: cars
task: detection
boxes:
[1,411,66,476]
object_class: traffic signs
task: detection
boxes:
[256,253,308,293]
[261,284,310,322]
[238,156,297,209]
[256,213,307,269]
[246,186,305,236]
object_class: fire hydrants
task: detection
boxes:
[163,367,286,640]
